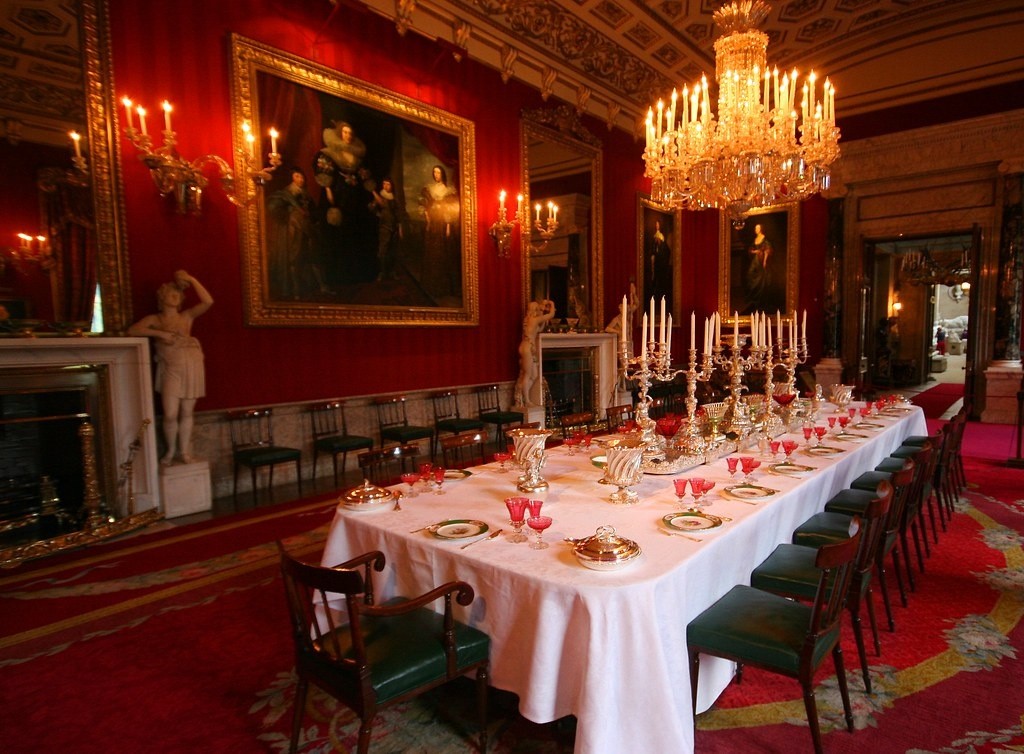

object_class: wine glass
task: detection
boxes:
[392,384,913,550]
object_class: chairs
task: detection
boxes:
[439,431,489,470]
[356,442,423,490]
[750,480,893,695]
[503,399,667,461]
[280,550,492,754]
[425,388,486,469]
[793,455,914,633]
[472,383,524,451]
[825,409,967,591]
[686,514,863,754]
[366,394,436,475]
[302,401,374,491]
[222,407,303,512]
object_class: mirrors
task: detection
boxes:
[1,2,133,337]
[518,104,604,331]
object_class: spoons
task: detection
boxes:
[686,508,732,521]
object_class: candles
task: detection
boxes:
[793,311,798,350]
[690,311,695,349]
[801,310,808,354]
[621,294,628,340]
[704,311,721,357]
[649,295,655,343]
[788,319,793,349]
[642,311,647,359]
[666,312,672,359]
[659,294,666,342]
[734,311,739,346]
[750,309,783,346]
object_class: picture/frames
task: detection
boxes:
[637,195,683,328]
[223,28,483,330]
[718,201,800,326]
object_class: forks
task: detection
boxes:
[657,527,702,543]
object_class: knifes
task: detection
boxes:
[461,529,503,549]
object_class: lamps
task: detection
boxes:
[641,2,842,231]
[7,232,56,279]
[121,96,283,218]
[488,190,560,260]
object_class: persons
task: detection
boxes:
[604,282,641,392]
[512,300,557,408]
[935,327,946,355]
[123,268,215,467]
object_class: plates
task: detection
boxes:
[430,469,473,479]
[886,408,911,413]
[663,512,722,531]
[835,434,868,440]
[808,447,845,456]
[769,463,814,473]
[724,485,775,498]
[854,423,885,430]
[428,519,488,538]
[870,413,899,420]
